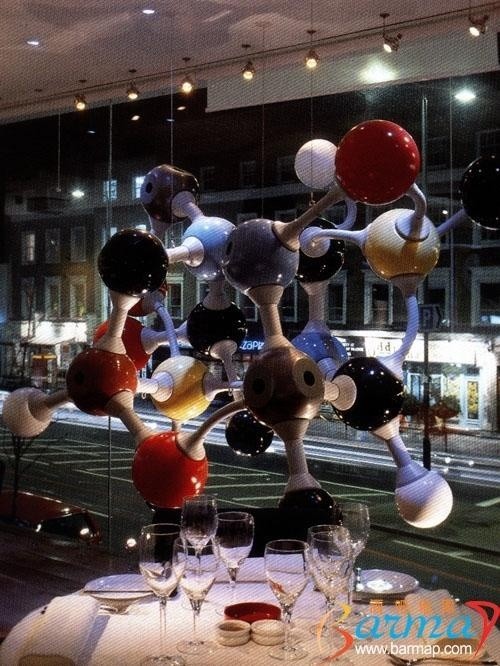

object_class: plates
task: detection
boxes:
[84,574,155,600]
[351,569,420,596]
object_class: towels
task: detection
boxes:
[405,588,487,661]
[19,594,100,666]
[177,551,266,582]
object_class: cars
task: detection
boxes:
[0,489,101,562]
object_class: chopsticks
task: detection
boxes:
[352,597,460,606]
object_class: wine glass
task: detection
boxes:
[139,491,371,665]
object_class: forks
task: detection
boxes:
[381,646,499,666]
